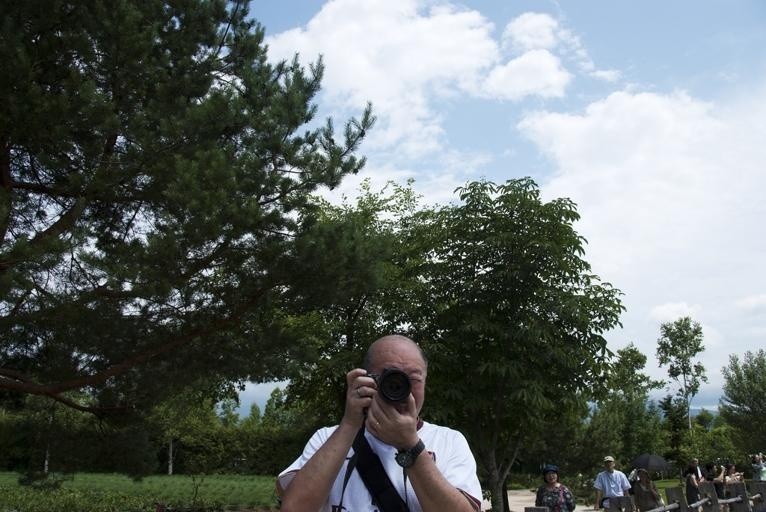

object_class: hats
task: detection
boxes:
[543,466,559,473]
[637,468,649,475]
[604,456,614,461]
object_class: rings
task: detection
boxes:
[356,388,361,395]
[373,422,380,430]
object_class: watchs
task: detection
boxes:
[393,437,425,468]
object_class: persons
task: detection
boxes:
[273,334,484,512]
[592,456,632,512]
[534,464,576,512]
[681,458,745,508]
[633,467,662,512]
[750,452,766,480]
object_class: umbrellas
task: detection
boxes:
[634,453,672,478]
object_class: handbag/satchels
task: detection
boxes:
[657,497,665,506]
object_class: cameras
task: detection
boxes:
[365,368,411,405]
